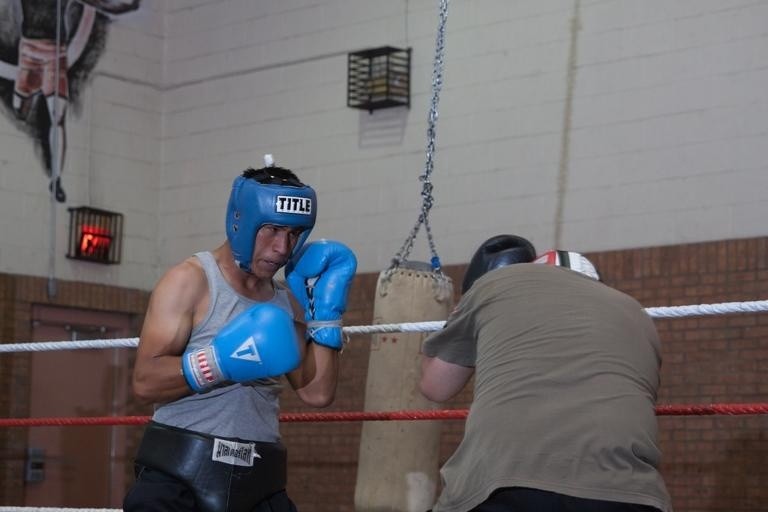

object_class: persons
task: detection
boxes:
[7,3,145,202]
[117,165,359,510]
[415,230,676,511]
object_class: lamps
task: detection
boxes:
[67,205,122,265]
[343,45,415,113]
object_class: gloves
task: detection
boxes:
[285,239,356,351]
[461,235,536,297]
[183,303,301,394]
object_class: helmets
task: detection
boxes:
[531,251,599,281]
[226,176,316,271]
